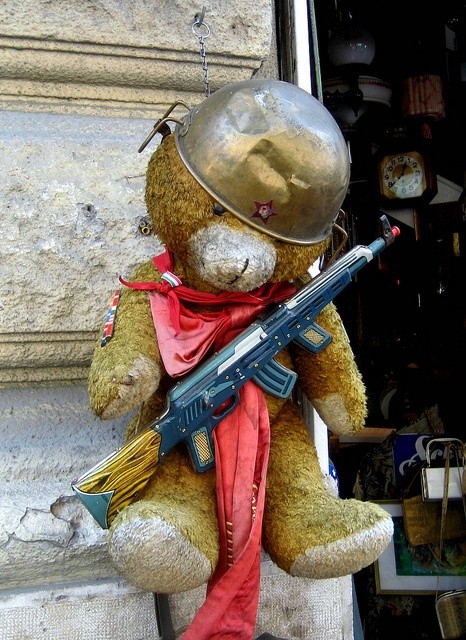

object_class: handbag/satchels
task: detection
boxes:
[401,496,466,547]
[435,589,466,640]
[420,467,464,503]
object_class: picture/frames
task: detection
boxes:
[365,499,466,597]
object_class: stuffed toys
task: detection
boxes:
[86,133,394,596]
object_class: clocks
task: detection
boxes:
[374,141,438,209]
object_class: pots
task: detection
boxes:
[140,78,350,271]
